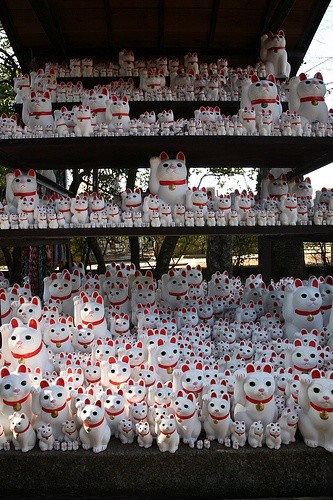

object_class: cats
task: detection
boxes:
[0,260,333,453]
[0,151,333,228]
[2,30,333,139]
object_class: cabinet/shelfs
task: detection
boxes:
[0,75,333,494]
